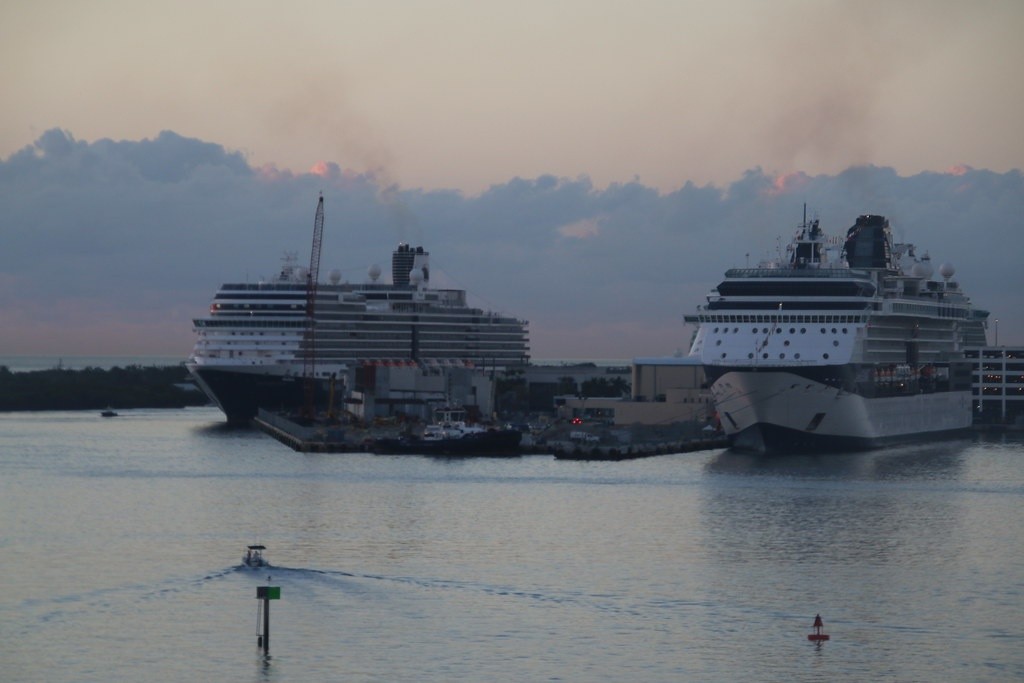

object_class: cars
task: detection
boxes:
[423,421,466,441]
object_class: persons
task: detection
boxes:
[245,550,260,561]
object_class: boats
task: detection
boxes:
[682,202,973,456]
[101,410,118,417]
[240,544,270,570]
[184,241,531,424]
[807,629,831,642]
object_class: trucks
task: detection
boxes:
[571,432,599,444]
